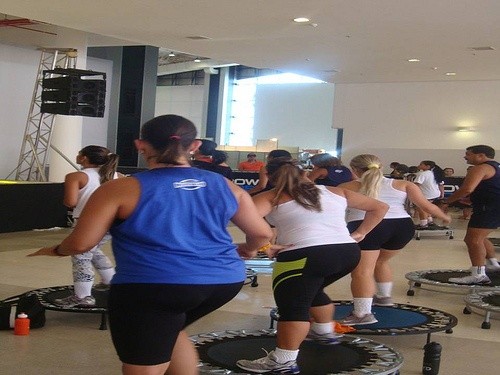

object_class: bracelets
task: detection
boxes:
[54,244,66,256]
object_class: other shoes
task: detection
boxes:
[414,224,428,229]
[427,221,434,226]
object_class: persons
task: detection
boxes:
[189,139,232,180]
[236,157,389,375]
[55,146,126,305]
[239,153,265,171]
[27,114,273,375]
[441,145,500,285]
[247,150,479,327]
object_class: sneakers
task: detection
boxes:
[236,348,301,375]
[53,294,96,307]
[301,329,341,346]
[371,293,394,307]
[448,274,492,285]
[338,310,378,326]
[94,283,111,292]
[469,264,500,273]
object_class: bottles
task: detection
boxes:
[422,341,442,375]
[14,312,30,335]
